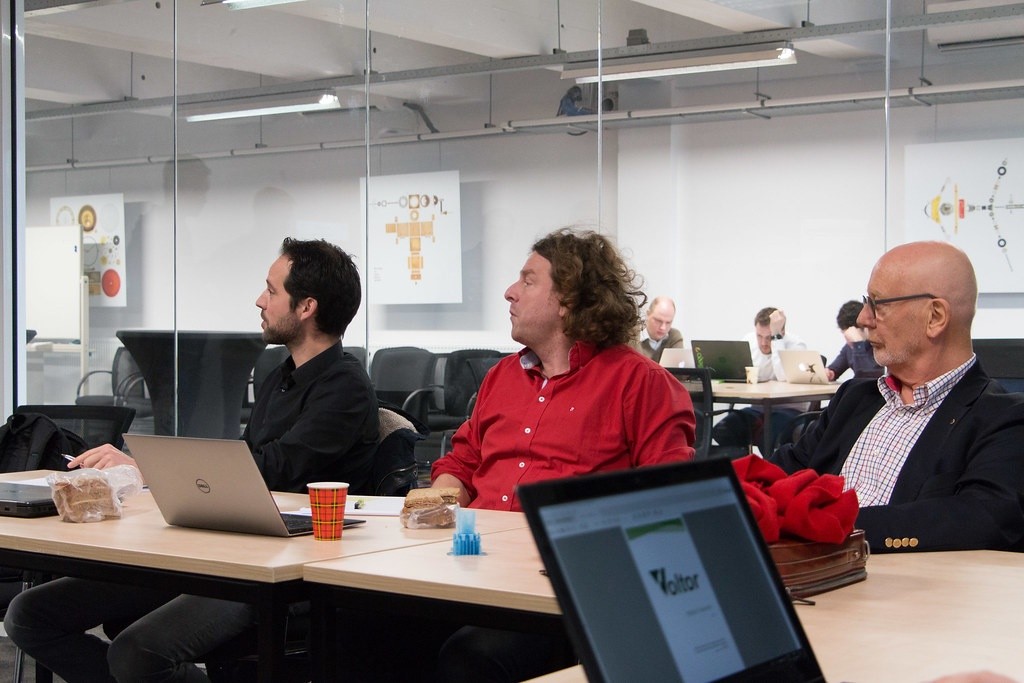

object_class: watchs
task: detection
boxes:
[771,334,782,340]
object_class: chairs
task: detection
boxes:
[711,355,826,433]
[14,339,1024,683]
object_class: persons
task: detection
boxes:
[767,241,1024,554]
[3,239,379,682]
[354,228,694,683]
[825,300,885,381]
[713,308,809,458]
[633,297,683,364]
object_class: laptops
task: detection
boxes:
[122,433,366,537]
[691,340,769,383]
[0,482,58,518]
[659,348,695,368]
[778,350,843,385]
[515,455,827,683]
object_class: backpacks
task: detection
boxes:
[0,410,90,472]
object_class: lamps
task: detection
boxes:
[200,0,305,11]
[561,40,796,84]
[183,89,340,122]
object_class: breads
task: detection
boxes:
[52,476,121,521]
[401,487,460,528]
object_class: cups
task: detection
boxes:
[745,367,759,384]
[306,482,349,542]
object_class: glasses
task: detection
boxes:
[862,293,939,320]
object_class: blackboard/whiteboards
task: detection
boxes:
[25,225,83,344]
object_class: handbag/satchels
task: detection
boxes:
[764,529,871,601]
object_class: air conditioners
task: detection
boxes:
[927,0,1024,51]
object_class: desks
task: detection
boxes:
[518,469,1024,683]
[710,380,840,456]
[304,523,561,683]
[1,470,532,682]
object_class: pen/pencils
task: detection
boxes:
[61,454,75,461]
[355,499,364,509]
[539,570,548,575]
